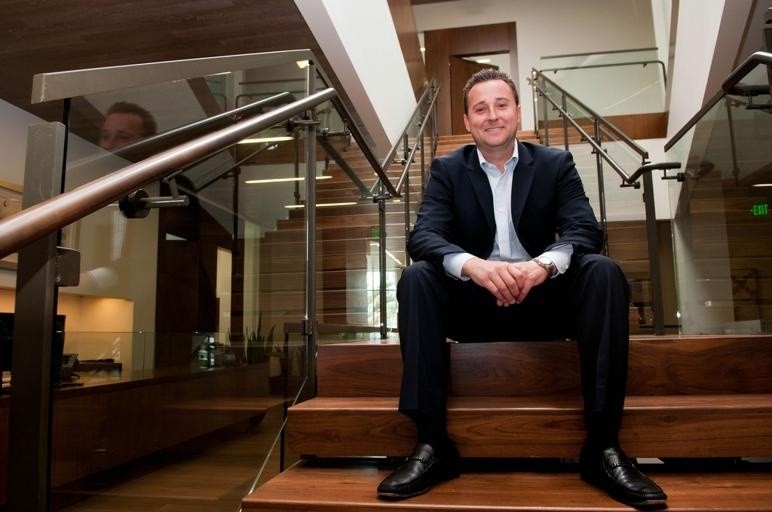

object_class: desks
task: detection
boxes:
[0,361,271,508]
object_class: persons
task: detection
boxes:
[375,69,670,507]
[99,102,158,161]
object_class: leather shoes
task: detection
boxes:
[579,439,668,506]
[377,444,461,497]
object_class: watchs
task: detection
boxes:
[532,255,557,278]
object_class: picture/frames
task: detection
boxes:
[0,178,82,273]
